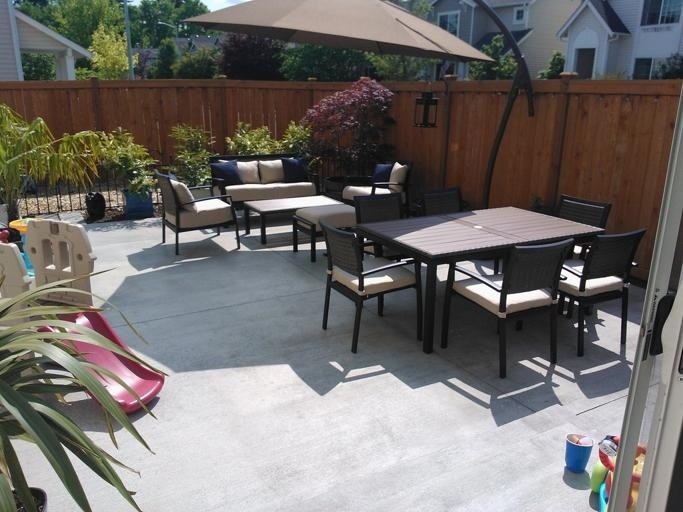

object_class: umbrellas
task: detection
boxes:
[178,0,535,209]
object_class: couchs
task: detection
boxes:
[203,152,320,211]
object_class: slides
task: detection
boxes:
[37,305,165,413]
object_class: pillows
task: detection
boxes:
[280,157,310,182]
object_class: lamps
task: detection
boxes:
[413,78,448,129]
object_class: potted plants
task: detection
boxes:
[103,126,158,219]
[0,262,169,512]
[299,75,401,202]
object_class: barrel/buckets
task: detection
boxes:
[565,434,594,473]
